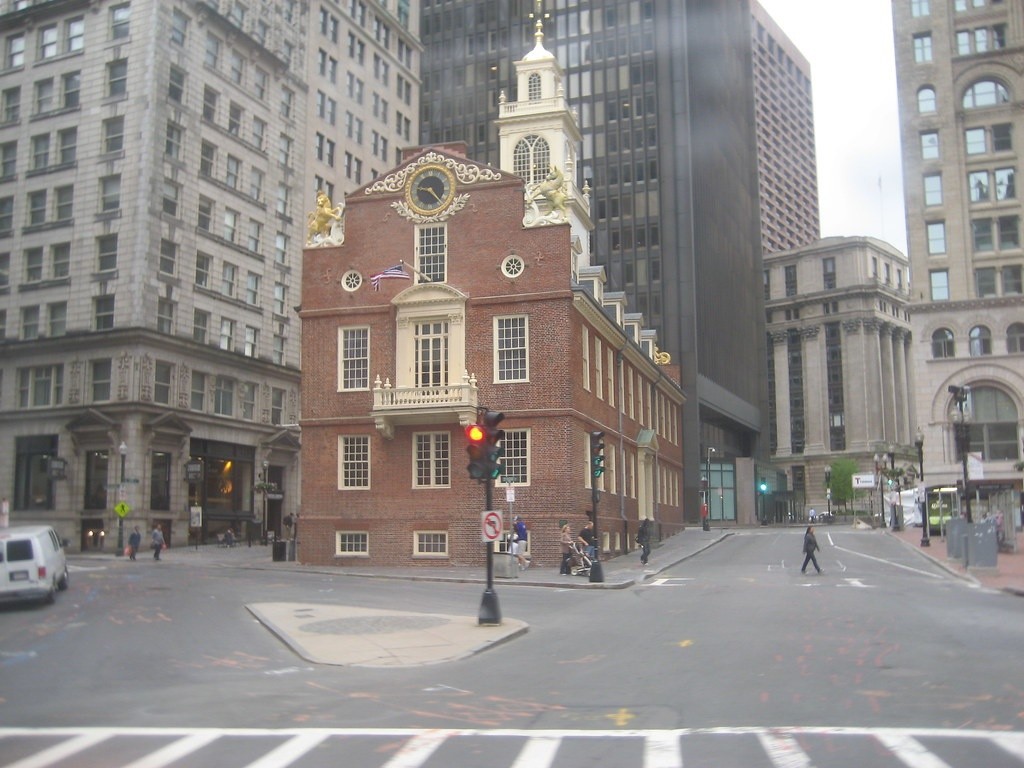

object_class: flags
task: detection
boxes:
[369,264,410,291]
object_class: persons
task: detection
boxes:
[129,526,141,560]
[801,527,824,575]
[152,524,165,561]
[559,524,573,576]
[283,513,294,540]
[809,508,815,523]
[512,515,530,571]
[638,518,651,566]
[578,521,594,576]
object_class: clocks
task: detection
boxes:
[405,163,456,218]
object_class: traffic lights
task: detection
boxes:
[478,408,505,481]
[465,425,486,480]
[591,430,607,477]
[760,484,767,491]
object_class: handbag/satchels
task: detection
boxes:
[124,546,133,556]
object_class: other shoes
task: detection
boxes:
[819,569,823,573]
[641,557,649,566]
[801,570,806,574]
[560,571,567,575]
[519,561,531,570]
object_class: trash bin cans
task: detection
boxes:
[273,541,286,561]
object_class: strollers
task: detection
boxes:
[567,543,593,579]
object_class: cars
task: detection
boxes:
[809,511,833,521]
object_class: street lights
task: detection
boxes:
[873,453,888,528]
[887,443,900,531]
[914,426,930,546]
[114,440,128,557]
[823,464,833,518]
[706,446,716,520]
[946,384,973,524]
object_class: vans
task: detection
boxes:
[0,523,70,607]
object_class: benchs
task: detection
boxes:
[216,532,240,548]
